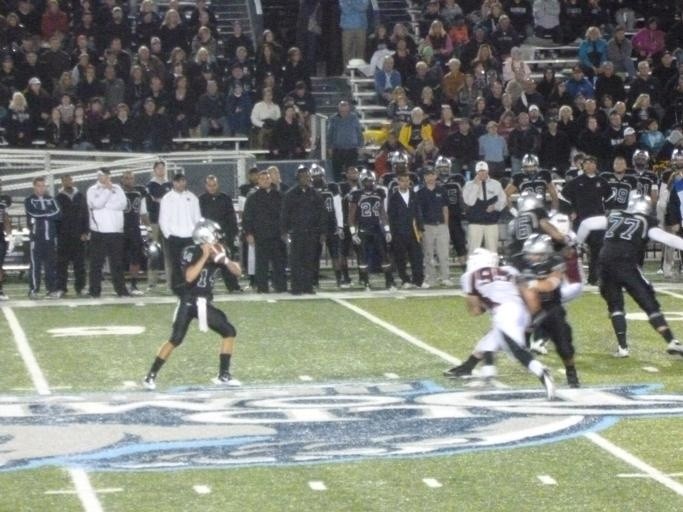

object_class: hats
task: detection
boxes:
[474,161,489,173]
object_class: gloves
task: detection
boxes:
[209,244,226,264]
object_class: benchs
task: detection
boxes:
[348,49,389,134]
[519,34,584,84]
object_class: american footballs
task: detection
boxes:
[208,244,227,266]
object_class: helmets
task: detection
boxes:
[522,233,555,267]
[522,148,683,175]
[467,248,498,272]
[193,219,227,248]
[625,193,653,217]
[295,149,452,191]
[517,191,545,213]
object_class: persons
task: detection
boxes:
[441,195,683,401]
[0,0,682,300]
[145,219,243,386]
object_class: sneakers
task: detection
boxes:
[337,280,455,293]
[0,282,156,301]
[443,340,682,401]
[216,371,242,387]
[143,372,155,391]
